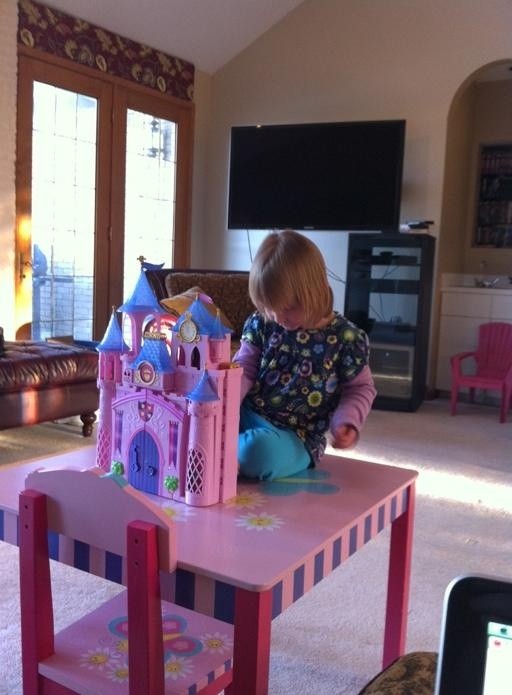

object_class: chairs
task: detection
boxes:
[449,321,511,423]
[18,464,235,695]
[144,267,260,362]
[359,651,438,695]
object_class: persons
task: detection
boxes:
[229,227,380,482]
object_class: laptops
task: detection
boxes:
[429,571,512,694]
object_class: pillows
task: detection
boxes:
[165,272,257,340]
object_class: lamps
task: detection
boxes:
[143,117,165,159]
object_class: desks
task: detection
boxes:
[0,441,419,695]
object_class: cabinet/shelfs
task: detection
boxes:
[344,233,436,411]
[434,291,512,409]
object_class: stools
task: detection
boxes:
[0,339,101,437]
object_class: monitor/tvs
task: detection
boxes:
[225,118,408,233]
